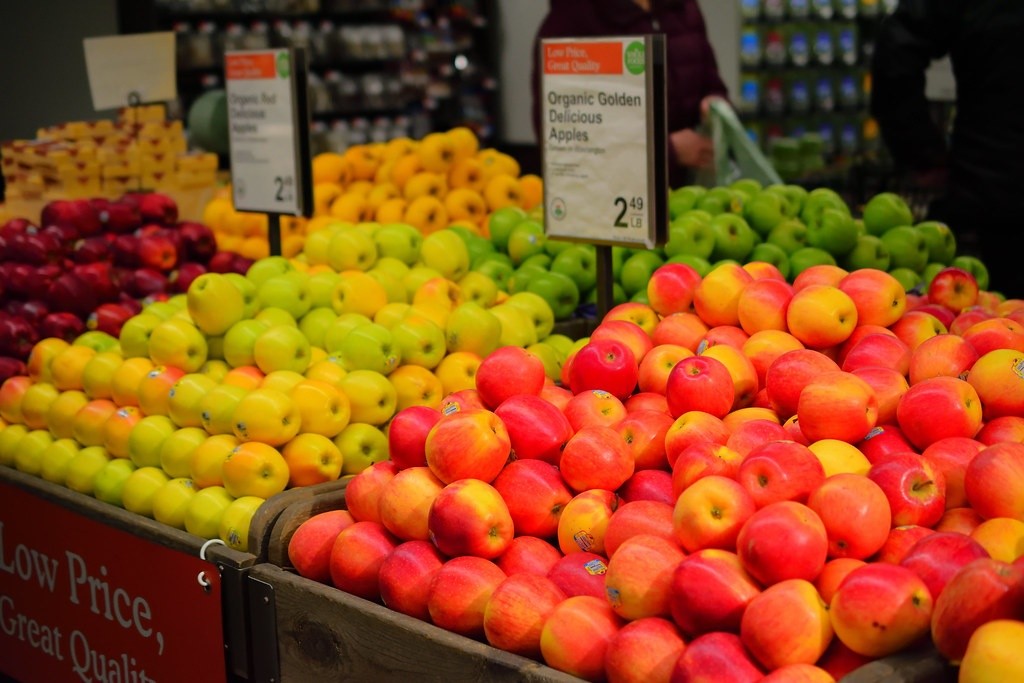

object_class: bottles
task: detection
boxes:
[167,0,494,159]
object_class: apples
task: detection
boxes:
[447,179,1004,317]
[286,263,1024,683]
[205,126,543,257]
[0,191,575,555]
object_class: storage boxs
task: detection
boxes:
[0,310,958,683]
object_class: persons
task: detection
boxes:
[871,0,1024,300]
[532,0,726,170]
